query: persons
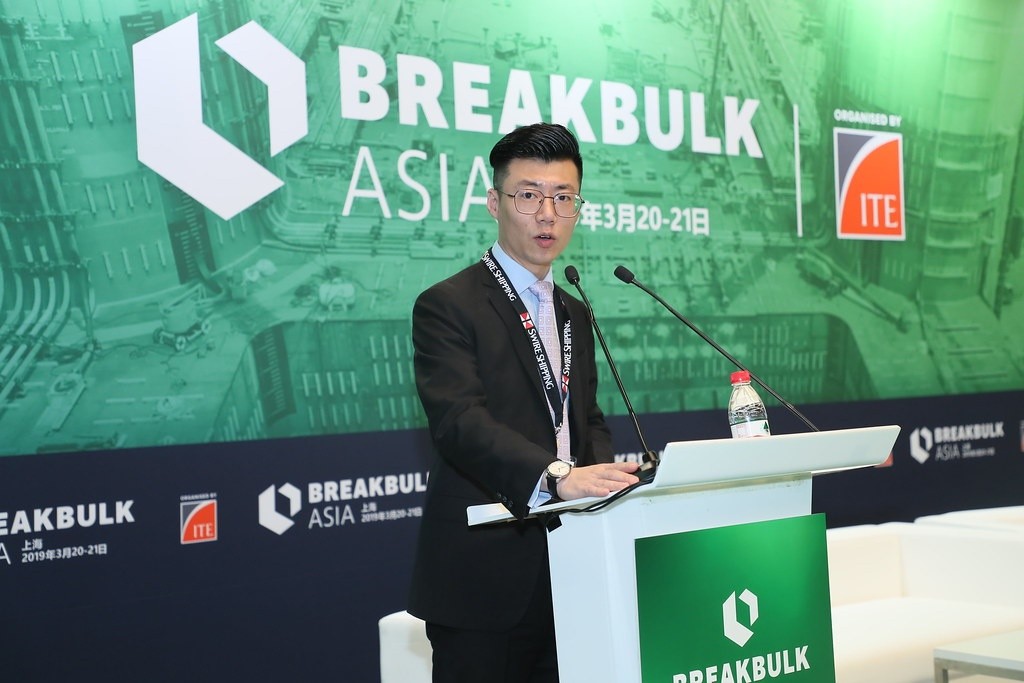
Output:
[405,125,639,683]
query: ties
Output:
[528,281,562,400]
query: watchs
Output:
[546,460,572,500]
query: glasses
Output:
[495,188,586,218]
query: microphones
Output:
[614,265,819,432]
[565,265,661,483]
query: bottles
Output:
[727,371,771,438]
